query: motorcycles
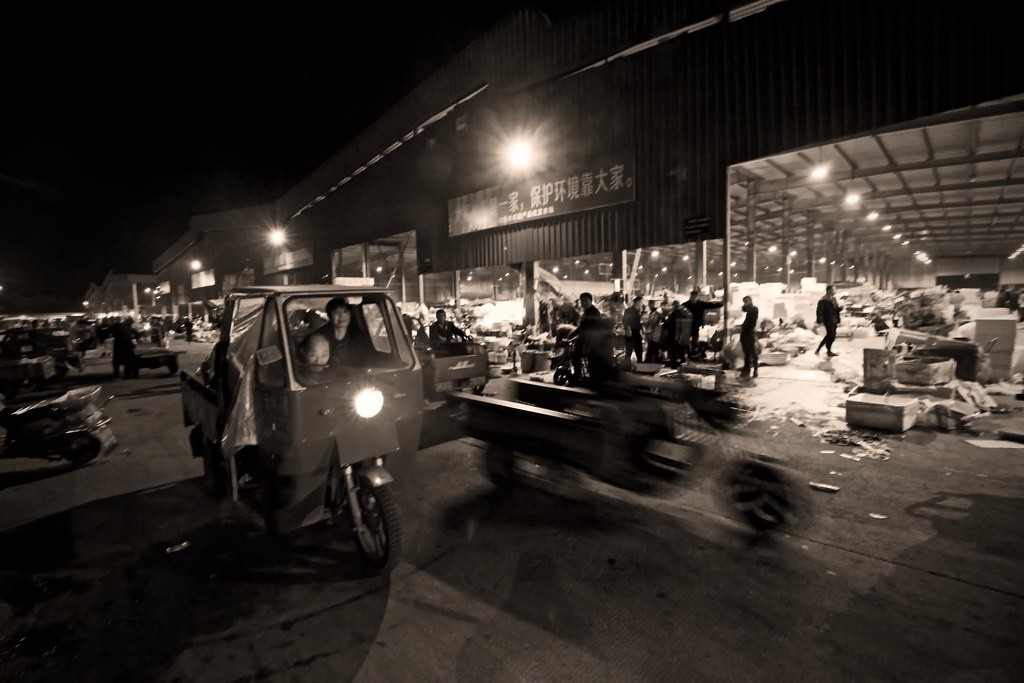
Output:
[177,283,428,574]
[127,326,190,379]
[370,314,492,401]
[0,383,120,466]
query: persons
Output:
[622,290,726,369]
[1,313,101,352]
[552,293,603,387]
[872,294,890,336]
[296,297,366,383]
[110,316,174,379]
[814,285,844,356]
[184,316,193,342]
[996,288,1024,323]
[430,310,466,356]
[735,296,758,379]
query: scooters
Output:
[548,342,637,400]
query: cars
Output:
[0,327,78,404]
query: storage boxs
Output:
[844,287,1019,430]
